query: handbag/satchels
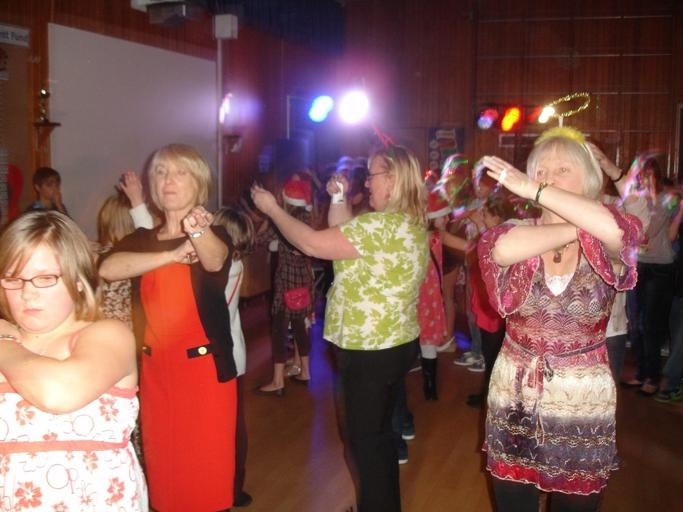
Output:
[283,286,312,310]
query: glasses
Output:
[365,169,388,182]
[0,272,62,290]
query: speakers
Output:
[215,14,237,40]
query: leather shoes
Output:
[620,379,643,389]
[638,386,660,398]
[253,364,310,397]
[234,491,252,506]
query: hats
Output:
[282,171,316,213]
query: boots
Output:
[418,355,438,402]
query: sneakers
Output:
[453,351,483,367]
[654,386,682,404]
[397,446,408,465]
[466,358,486,372]
[400,421,415,441]
